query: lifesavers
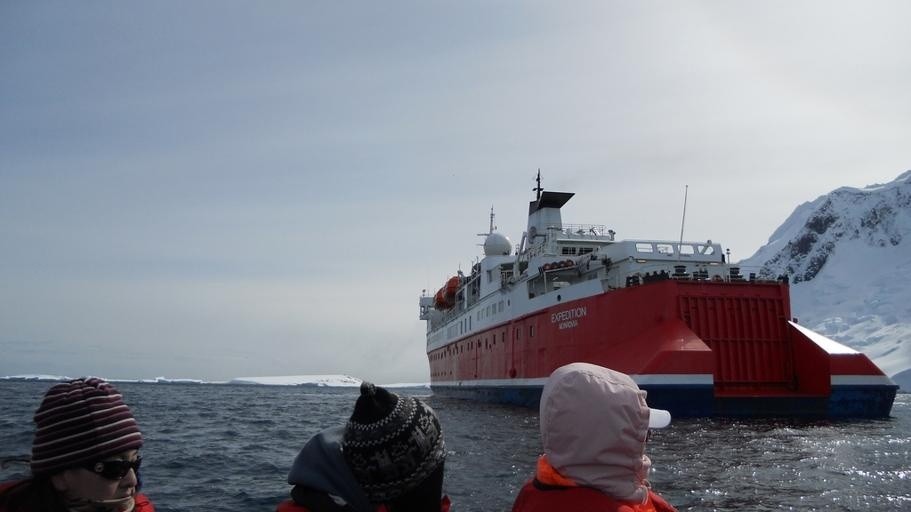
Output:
[711,275,722,281]
[545,259,574,270]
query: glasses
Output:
[80,457,143,481]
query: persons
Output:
[0,374,155,510]
[513,361,679,511]
[285,380,453,512]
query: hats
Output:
[29,375,144,478]
[341,386,448,503]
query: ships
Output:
[419,168,900,418]
